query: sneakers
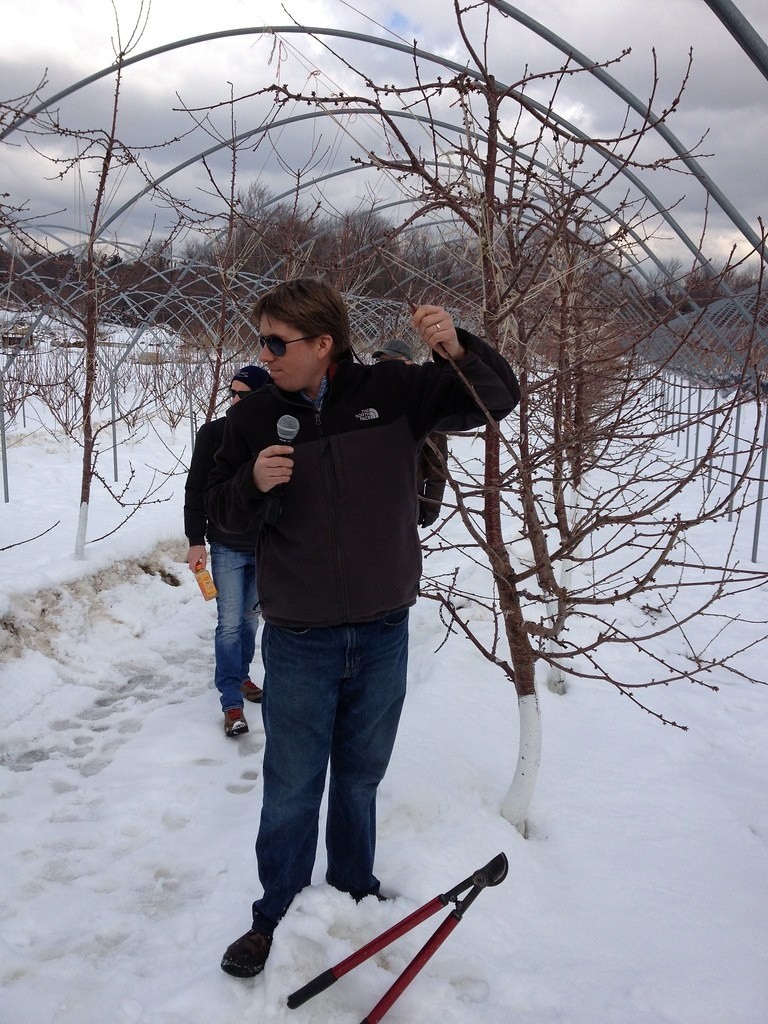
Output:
[224,707,249,738]
[221,913,274,978]
[326,872,386,903]
[240,680,263,703]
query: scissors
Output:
[285,852,512,1024]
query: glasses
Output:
[228,388,251,400]
[259,332,319,356]
[375,358,410,364]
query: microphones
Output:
[265,415,300,525]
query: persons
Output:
[372,339,448,529]
[201,279,519,977]
[184,366,274,736]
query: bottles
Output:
[195,563,218,602]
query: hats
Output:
[230,365,275,393]
[371,340,414,363]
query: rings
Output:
[435,323,441,333]
